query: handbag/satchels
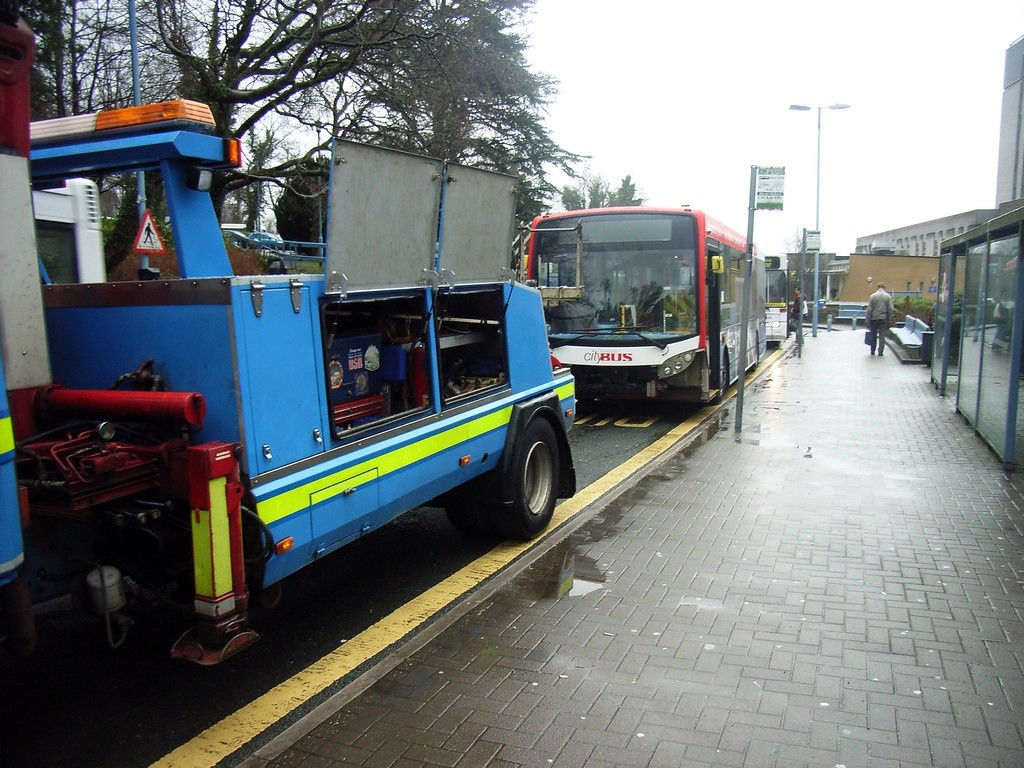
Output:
[865,327,873,345]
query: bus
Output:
[523,205,767,406]
[764,254,788,347]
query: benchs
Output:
[833,305,867,325]
[889,314,932,360]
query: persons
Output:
[830,285,839,298]
[865,282,893,355]
[791,286,809,346]
[991,285,1009,352]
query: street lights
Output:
[788,104,852,339]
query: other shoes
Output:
[879,353,884,357]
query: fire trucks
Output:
[0,0,578,668]
